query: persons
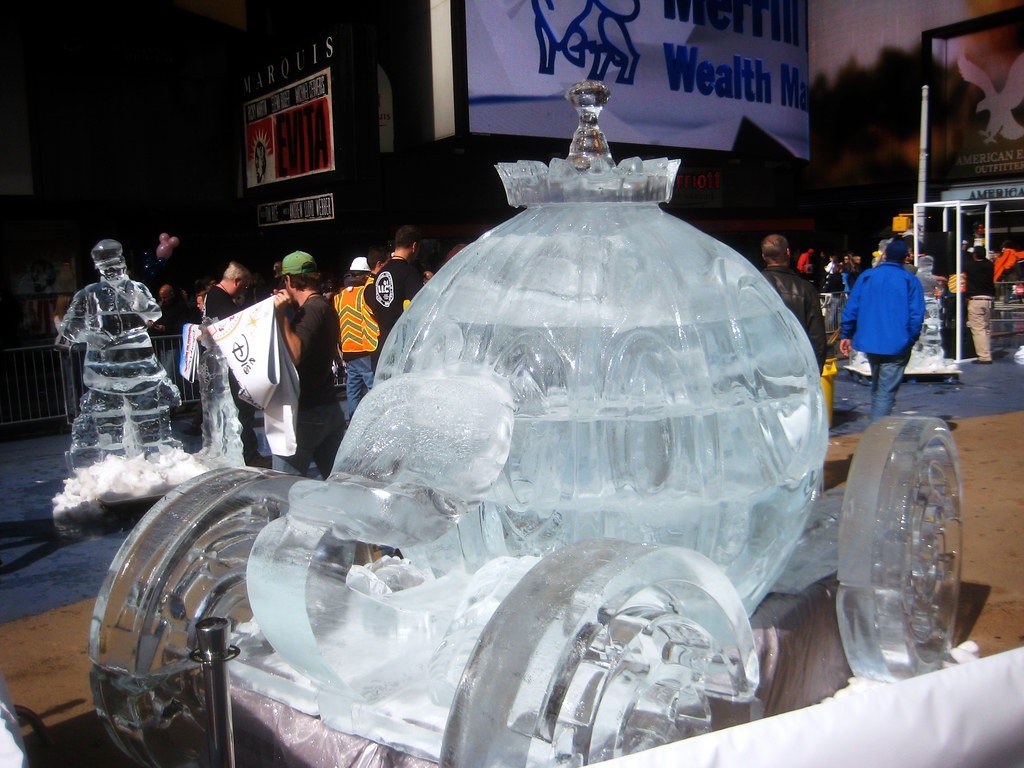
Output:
[206,262,271,466]
[798,241,995,363]
[375,225,421,362]
[758,235,826,377]
[840,241,925,425]
[271,251,347,481]
[334,257,375,420]
[147,246,434,437]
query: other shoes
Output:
[180,427,202,435]
[972,360,992,364]
[246,453,272,468]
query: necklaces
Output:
[220,283,228,294]
[307,293,322,298]
[392,256,406,261]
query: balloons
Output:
[131,233,180,279]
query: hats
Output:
[886,240,909,259]
[275,250,317,278]
[350,257,371,271]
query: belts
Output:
[970,298,991,300]
